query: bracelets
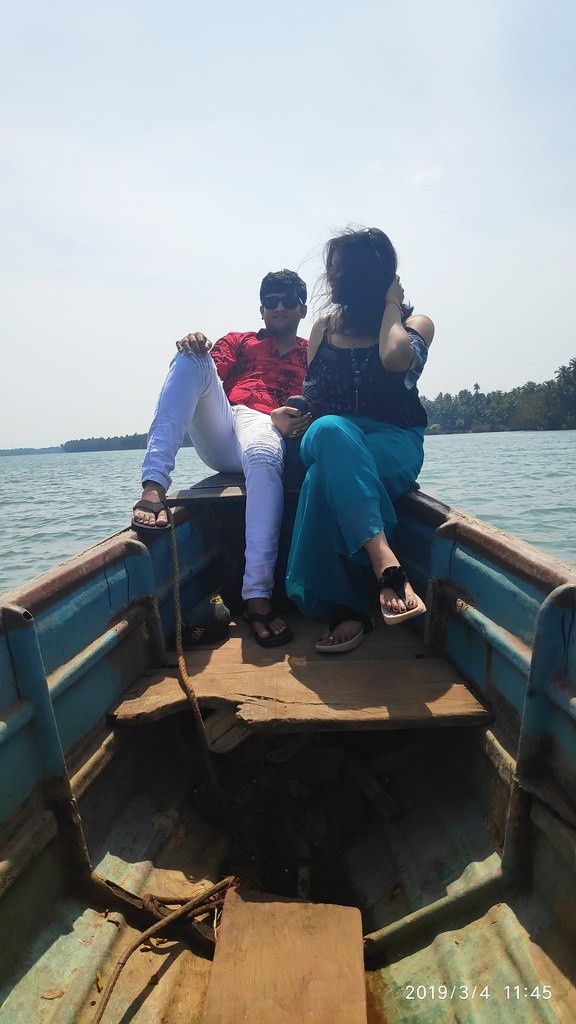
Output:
[385,301,404,319]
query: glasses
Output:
[263,295,304,309]
[355,226,386,270]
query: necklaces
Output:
[345,327,375,416]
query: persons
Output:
[272,227,436,655]
[131,269,310,649]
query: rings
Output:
[293,429,298,435]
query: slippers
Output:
[377,565,427,625]
[315,612,375,652]
[242,608,292,648]
[131,499,172,533]
